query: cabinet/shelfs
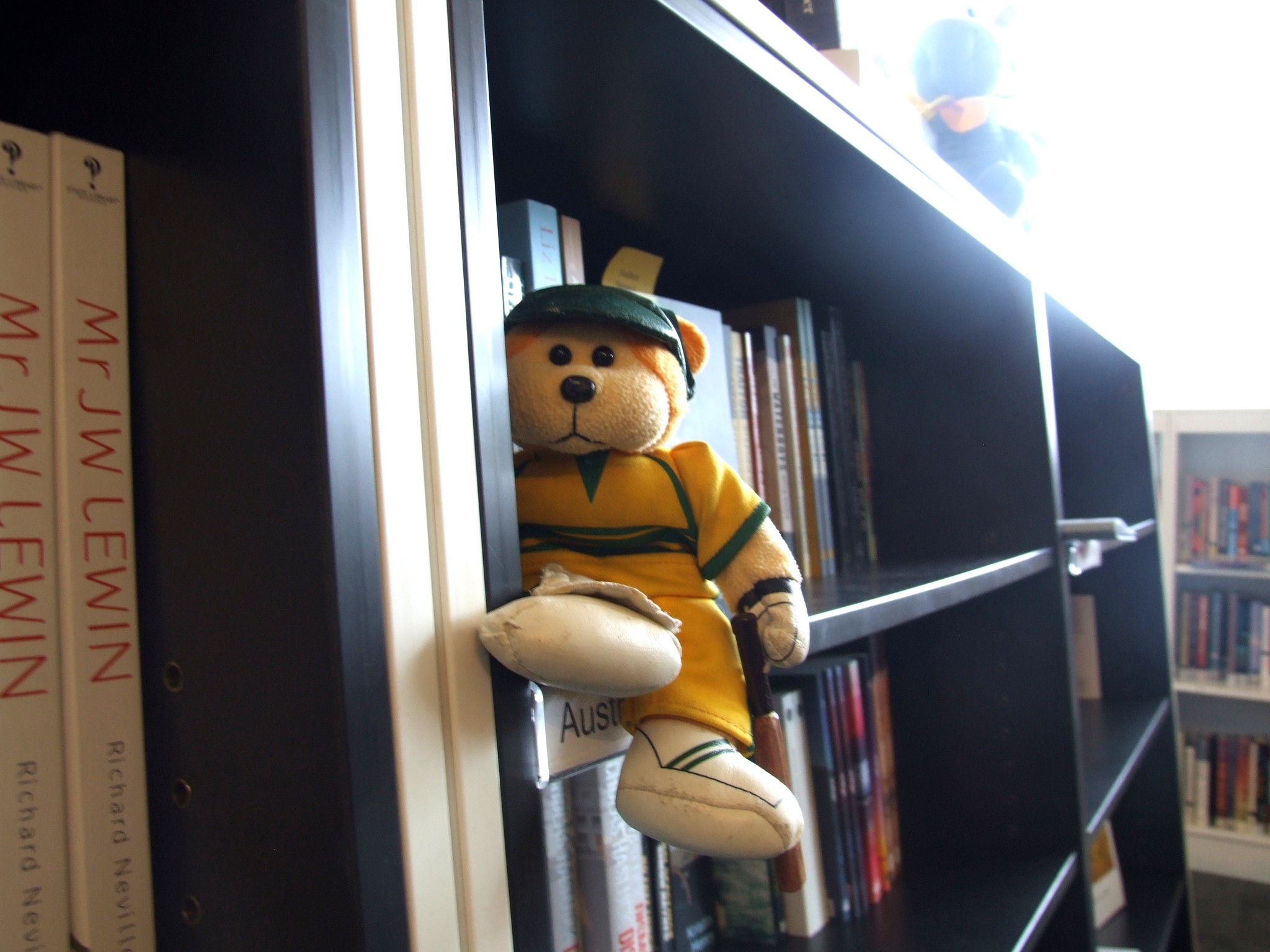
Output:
[0,0,1270,952]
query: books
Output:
[1179,589,1270,680]
[1089,820,1126,928]
[1183,731,1270,836]
[0,120,156,952]
[1070,596,1103,701]
[1180,476,1270,564]
[539,756,789,952]
[753,653,900,938]
[497,199,874,581]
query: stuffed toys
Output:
[478,283,810,859]
[910,8,1038,217]
